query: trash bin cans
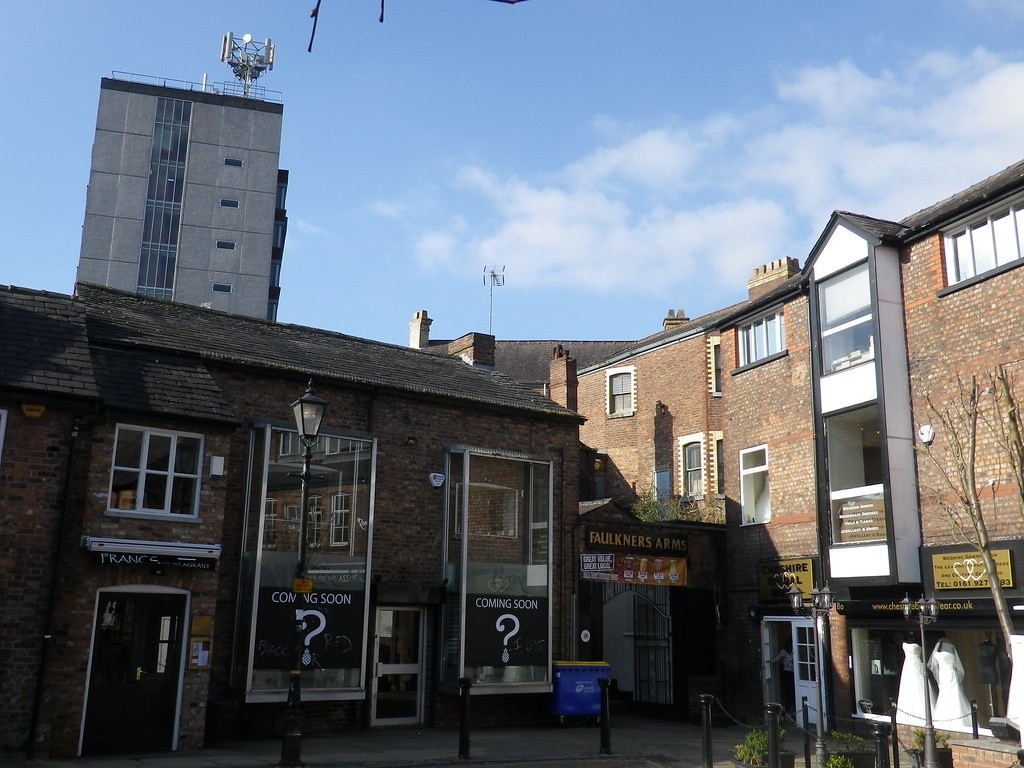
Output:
[552,661,612,727]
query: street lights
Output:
[279,377,329,768]
[788,578,836,768]
[902,590,940,768]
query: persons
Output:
[977,640,1000,683]
[895,632,936,725]
[927,638,980,728]
[768,636,795,712]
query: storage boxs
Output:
[837,500,887,540]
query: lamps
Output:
[988,716,1020,742]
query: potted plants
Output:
[723,728,876,768]
[905,728,955,768]
